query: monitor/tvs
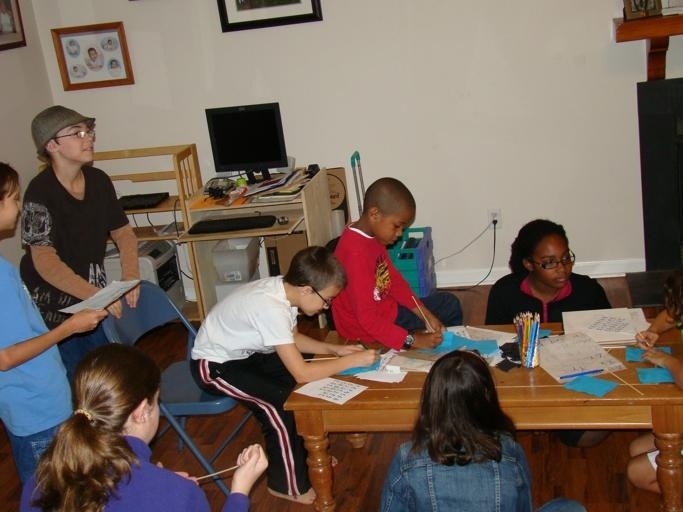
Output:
[204,101,288,185]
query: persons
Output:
[485,220,611,448]
[0,161,109,487]
[381,348,587,512]
[189,245,379,501]
[623,266,683,494]
[20,344,268,512]
[18,106,140,333]
[331,177,461,348]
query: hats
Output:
[32,105,95,156]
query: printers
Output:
[102,239,181,293]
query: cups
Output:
[517,339,542,368]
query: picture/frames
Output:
[50,22,135,91]
[623,0,663,22]
[0,0,28,51]
[217,0,324,33]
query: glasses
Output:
[531,249,576,268]
[51,130,94,138]
[302,286,331,309]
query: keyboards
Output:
[188,215,276,235]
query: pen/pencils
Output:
[636,327,651,348]
[560,369,603,379]
[513,311,540,368]
[357,339,370,350]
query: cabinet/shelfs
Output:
[38,143,203,324]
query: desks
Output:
[184,167,334,330]
[282,328,683,512]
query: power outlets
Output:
[487,208,503,228]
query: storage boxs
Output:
[214,272,260,302]
[209,236,259,282]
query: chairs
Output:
[100,278,255,498]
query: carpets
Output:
[624,271,669,308]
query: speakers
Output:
[277,156,296,172]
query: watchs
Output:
[403,331,414,349]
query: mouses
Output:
[278,216,289,225]
